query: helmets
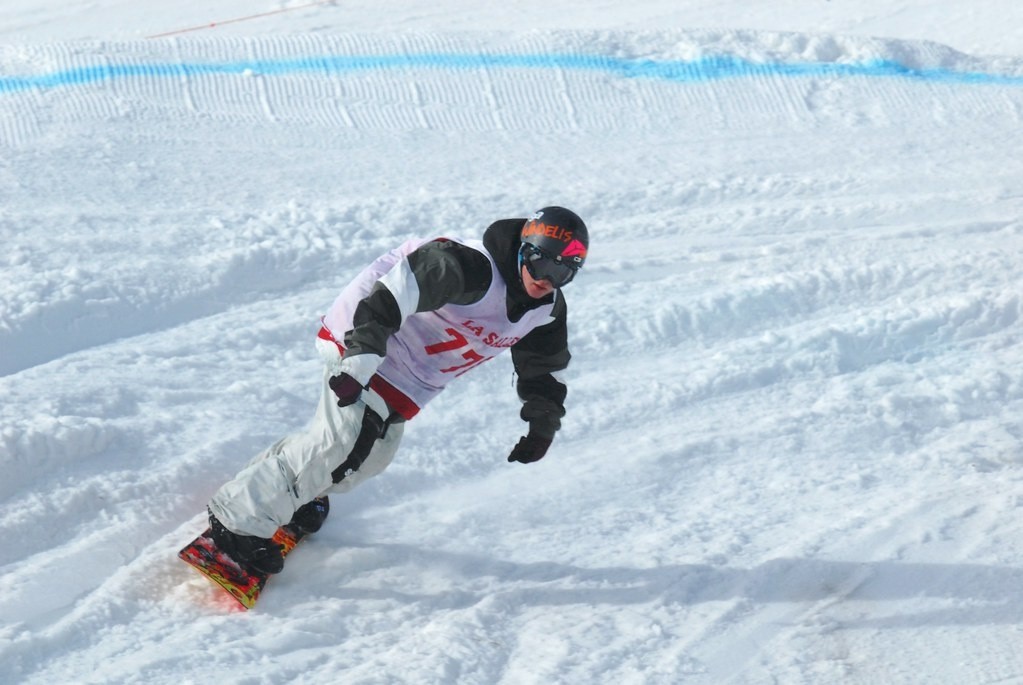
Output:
[519,206,589,268]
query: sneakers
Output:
[290,493,330,534]
[209,512,284,575]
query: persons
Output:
[205,206,588,576]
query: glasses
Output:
[516,242,577,289]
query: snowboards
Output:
[175,494,332,613]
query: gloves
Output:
[328,373,364,407]
[508,432,552,465]
